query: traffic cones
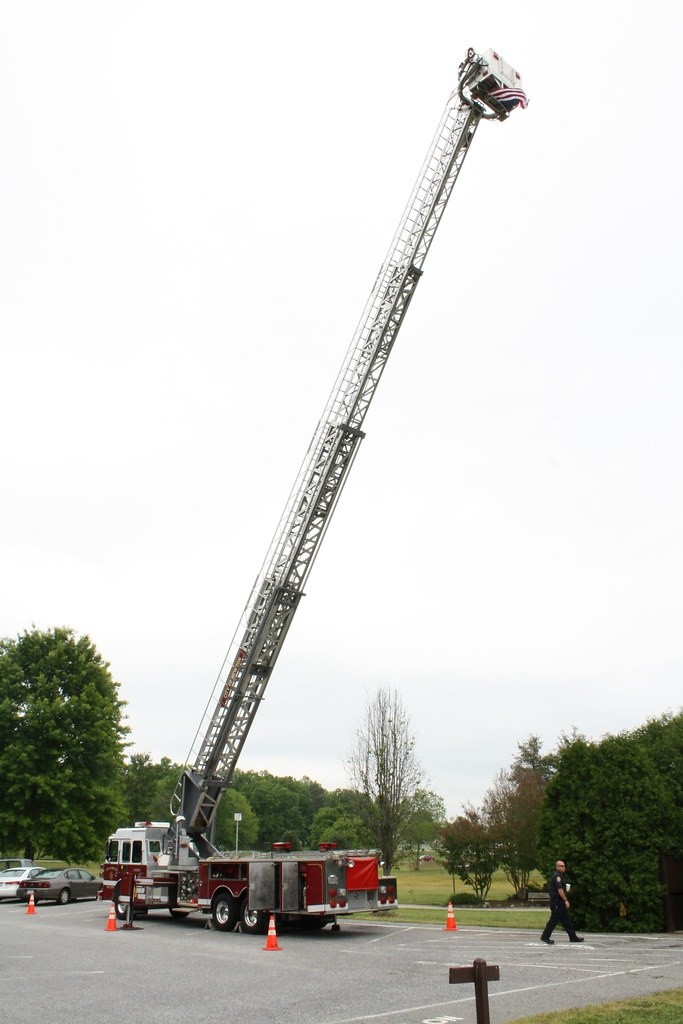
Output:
[104,903,118,931]
[262,915,283,951]
[24,892,37,915]
[442,902,459,931]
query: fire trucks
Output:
[92,42,530,936]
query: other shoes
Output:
[540,937,554,945]
[570,935,584,942]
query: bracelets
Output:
[564,899,568,901]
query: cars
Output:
[17,868,104,904]
[419,855,436,862]
[0,867,47,902]
[347,849,377,856]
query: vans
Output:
[0,859,36,872]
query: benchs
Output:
[528,892,550,907]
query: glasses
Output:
[558,865,565,867]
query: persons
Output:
[540,861,584,945]
